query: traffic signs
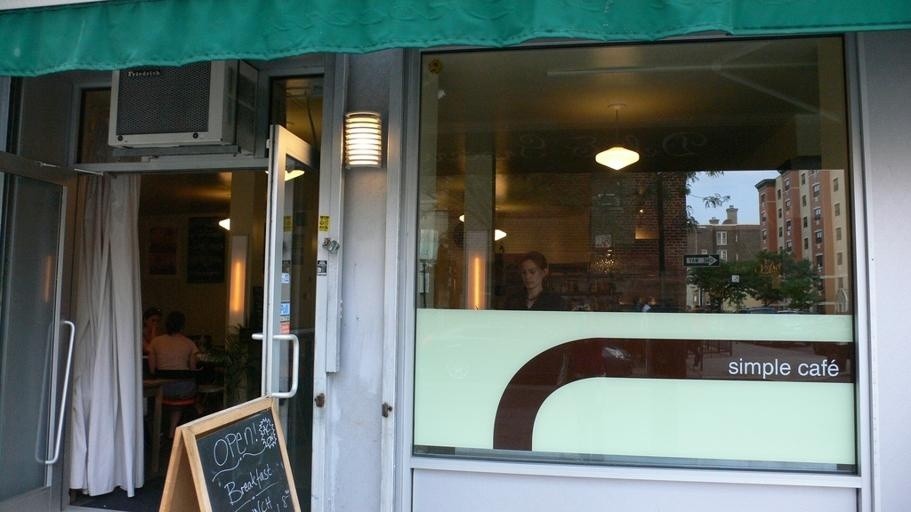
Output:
[684,253,720,268]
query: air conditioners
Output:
[108,56,259,157]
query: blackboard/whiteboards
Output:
[182,395,304,512]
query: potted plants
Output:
[685,251,827,312]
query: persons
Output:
[498,250,567,310]
[142,304,206,442]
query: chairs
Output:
[143,356,223,446]
